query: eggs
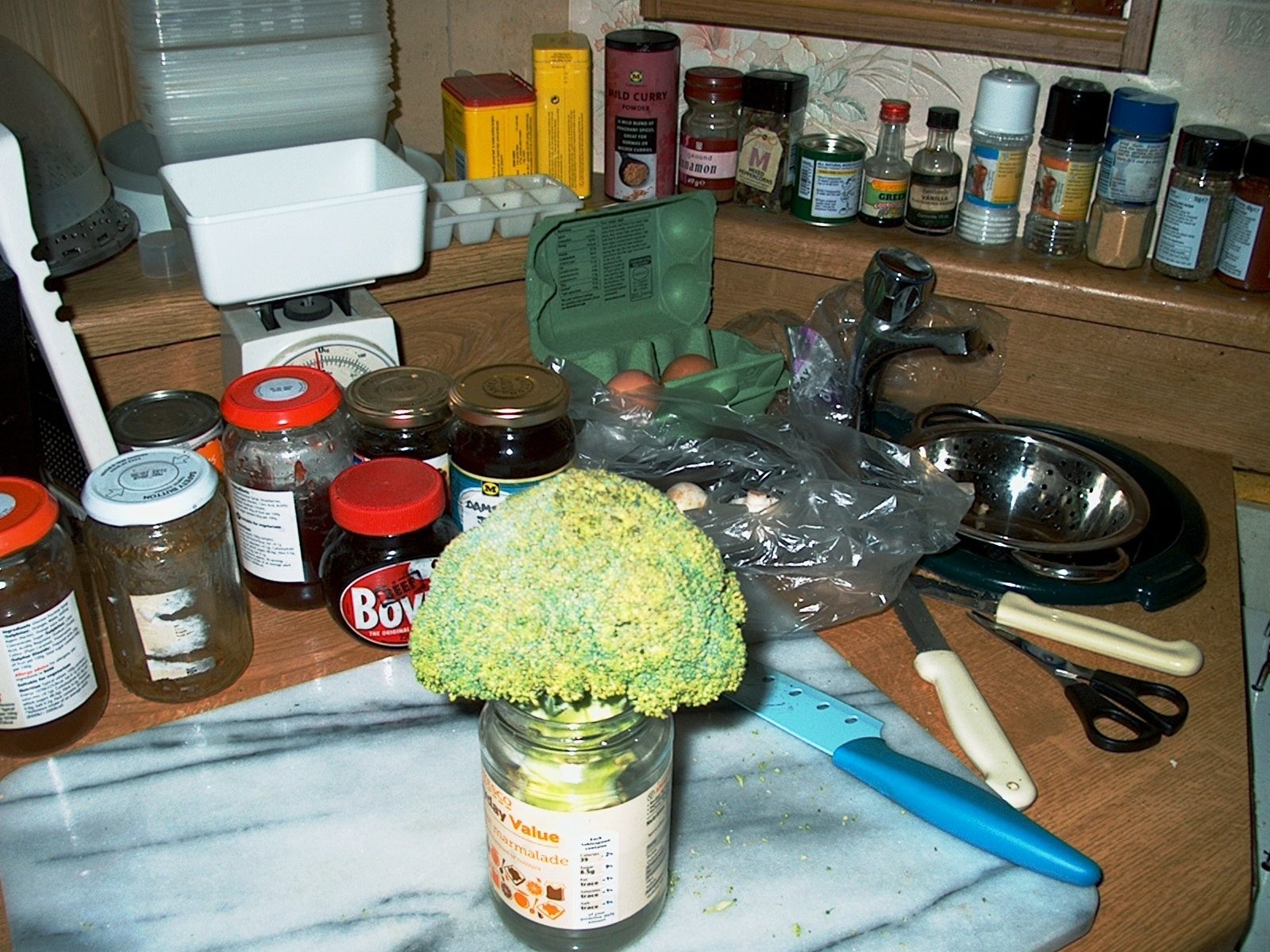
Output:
[603,350,718,416]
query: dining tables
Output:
[0,419,1263,948]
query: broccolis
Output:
[410,461,748,823]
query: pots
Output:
[903,403,1152,583]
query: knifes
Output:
[909,572,1206,676]
[892,578,1037,811]
[721,659,1105,887]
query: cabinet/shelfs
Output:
[63,170,1269,478]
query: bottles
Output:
[857,67,1270,291]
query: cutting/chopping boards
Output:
[3,566,1101,952]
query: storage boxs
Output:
[120,0,421,306]
